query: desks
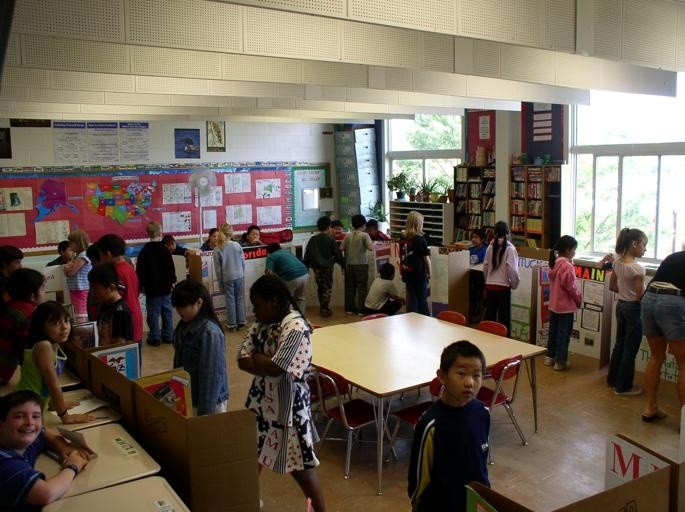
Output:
[307,312,548,495]
[0,356,194,512]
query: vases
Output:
[448,189,454,202]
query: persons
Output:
[1,211,431,512]
[481,219,522,339]
[406,338,491,512]
[605,227,648,396]
[638,248,685,423]
[465,231,489,264]
[543,235,583,372]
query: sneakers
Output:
[553,360,571,370]
[614,388,641,395]
[224,324,237,329]
[543,356,554,366]
[320,309,331,318]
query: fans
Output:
[187,166,217,248]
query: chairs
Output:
[307,378,336,428]
[475,355,528,466]
[478,321,508,337]
[383,377,444,463]
[437,310,466,326]
[315,366,398,479]
[361,314,388,321]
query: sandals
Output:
[642,410,667,422]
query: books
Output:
[452,161,550,248]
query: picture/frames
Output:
[290,162,331,233]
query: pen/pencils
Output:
[96,416,109,419]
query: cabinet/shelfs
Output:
[389,201,454,246]
[508,164,573,249]
[453,165,495,247]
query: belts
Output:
[647,285,681,295]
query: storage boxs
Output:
[334,128,380,221]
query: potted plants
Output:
[388,173,410,199]
[406,188,417,201]
[439,186,447,202]
[431,191,440,202]
[417,177,436,202]
[366,198,389,236]
